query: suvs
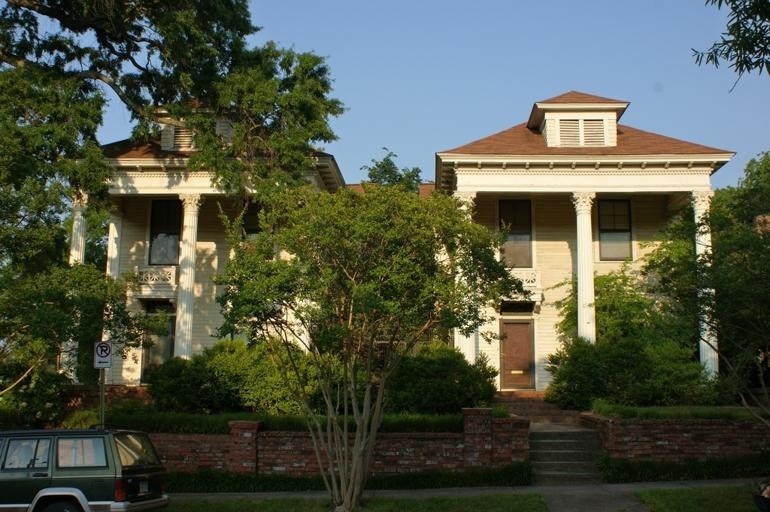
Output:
[0,428,171,512]
